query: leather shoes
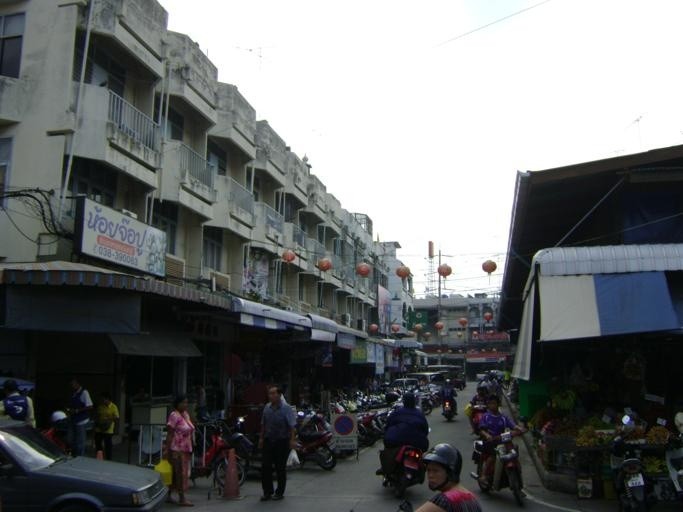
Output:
[262,493,283,501]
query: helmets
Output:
[445,379,451,385]
[423,443,462,482]
[51,411,67,422]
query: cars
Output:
[0,416,170,511]
[476,370,505,382]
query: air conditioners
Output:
[202,266,231,296]
[144,253,186,288]
[318,307,328,317]
[276,293,291,307]
[298,301,312,314]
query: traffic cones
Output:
[95,449,104,461]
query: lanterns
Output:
[282,251,498,353]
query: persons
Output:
[376,391,429,474]
[215,382,225,409]
[1,373,149,460]
[259,384,298,501]
[165,395,195,505]
[438,370,521,482]
[197,382,206,418]
[413,442,480,511]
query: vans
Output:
[419,363,465,391]
[402,372,443,382]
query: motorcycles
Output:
[655,415,681,511]
[438,388,458,422]
[471,416,528,509]
[288,378,442,458]
[252,422,336,470]
[466,383,504,438]
[348,501,414,512]
[596,413,649,511]
[195,414,278,481]
[139,412,247,489]
[377,402,430,498]
